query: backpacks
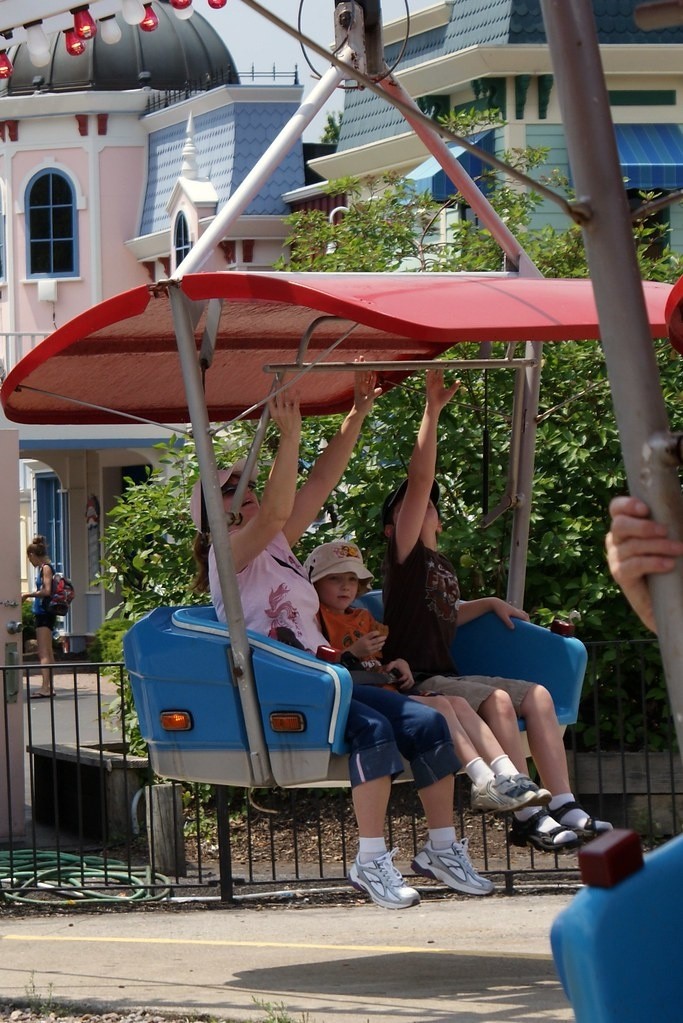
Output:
[40,563,74,616]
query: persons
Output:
[603,273,683,634]
[21,535,58,699]
[188,354,497,910]
[378,368,613,856]
[302,539,553,812]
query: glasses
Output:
[222,481,256,496]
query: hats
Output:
[303,541,375,600]
[190,458,258,534]
[382,477,440,524]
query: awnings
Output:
[567,123,683,194]
[396,129,495,206]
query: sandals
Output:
[545,801,609,840]
[508,811,582,852]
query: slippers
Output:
[30,691,56,698]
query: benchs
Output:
[26,744,151,844]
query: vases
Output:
[66,635,86,653]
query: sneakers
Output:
[411,838,494,895]
[470,775,536,814]
[512,774,552,812]
[348,847,420,909]
